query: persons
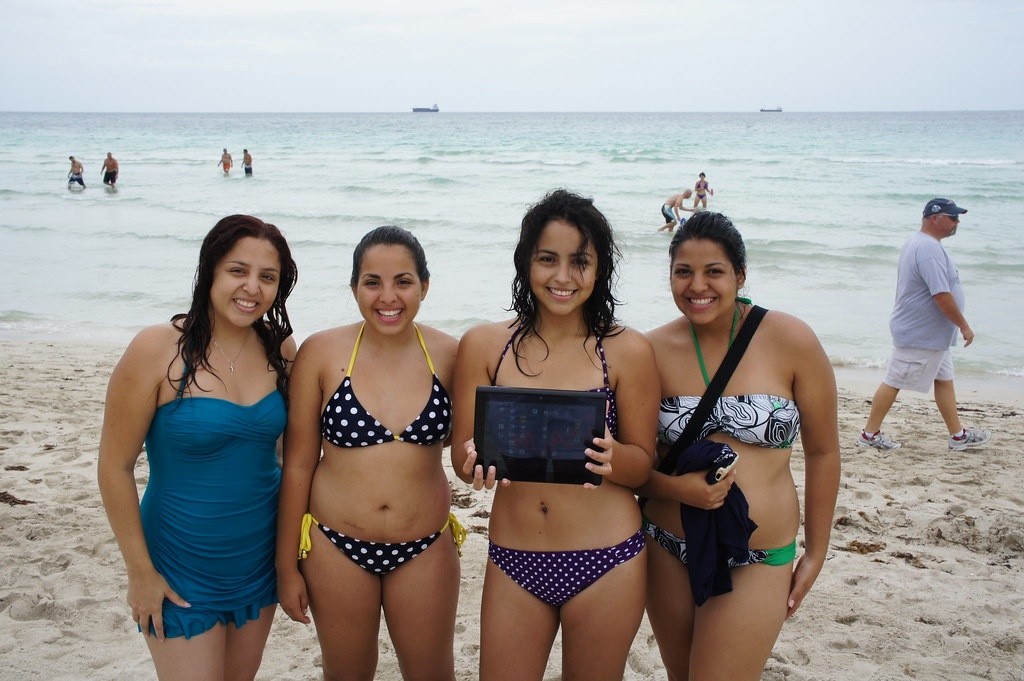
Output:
[218,148,232,174]
[451,187,661,681]
[693,172,714,208]
[100,152,118,188]
[856,198,994,452]
[98,215,298,681]
[643,213,838,681]
[241,149,252,175]
[68,156,87,189]
[277,227,462,681]
[658,189,695,232]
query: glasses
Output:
[942,213,959,220]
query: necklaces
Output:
[212,327,252,372]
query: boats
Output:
[412,104,440,112]
[760,107,782,112]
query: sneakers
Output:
[857,429,901,450]
[948,427,991,449]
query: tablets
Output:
[472,386,607,487]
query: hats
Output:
[922,198,968,217]
